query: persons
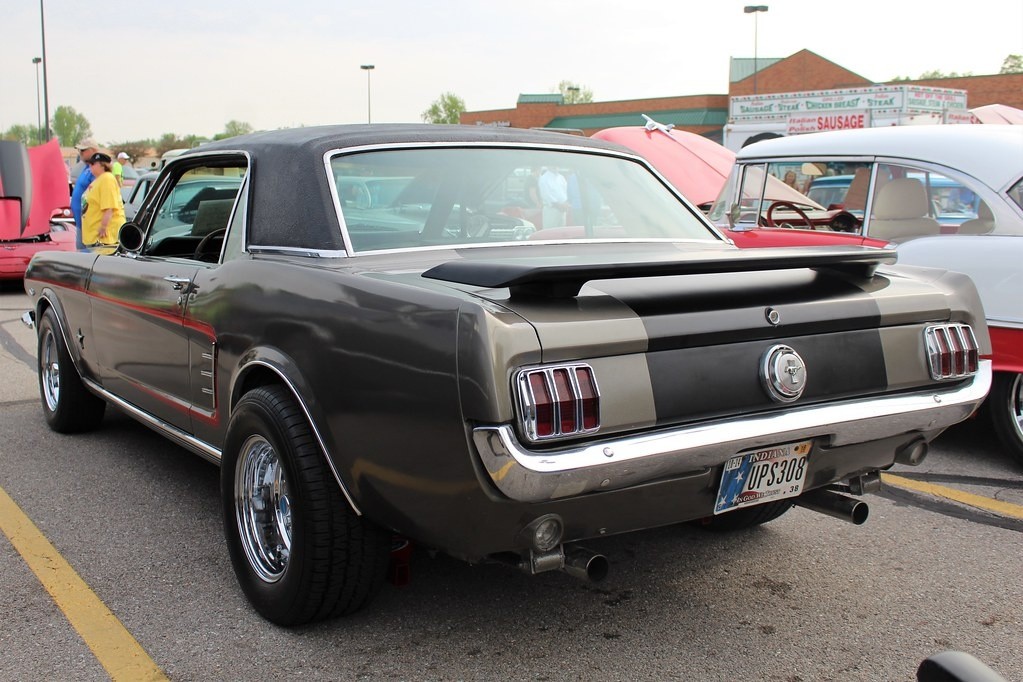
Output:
[523,162,573,228]
[69,139,96,250]
[782,170,811,195]
[110,151,131,204]
[81,154,127,248]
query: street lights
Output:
[31,56,42,144]
[744,4,770,97]
[360,65,375,124]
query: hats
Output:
[85,153,110,163]
[75,143,98,150]
[118,152,129,159]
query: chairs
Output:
[956,197,995,237]
[867,178,941,245]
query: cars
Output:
[22,125,992,638]
[0,134,80,278]
[533,106,1023,456]
[61,146,534,250]
